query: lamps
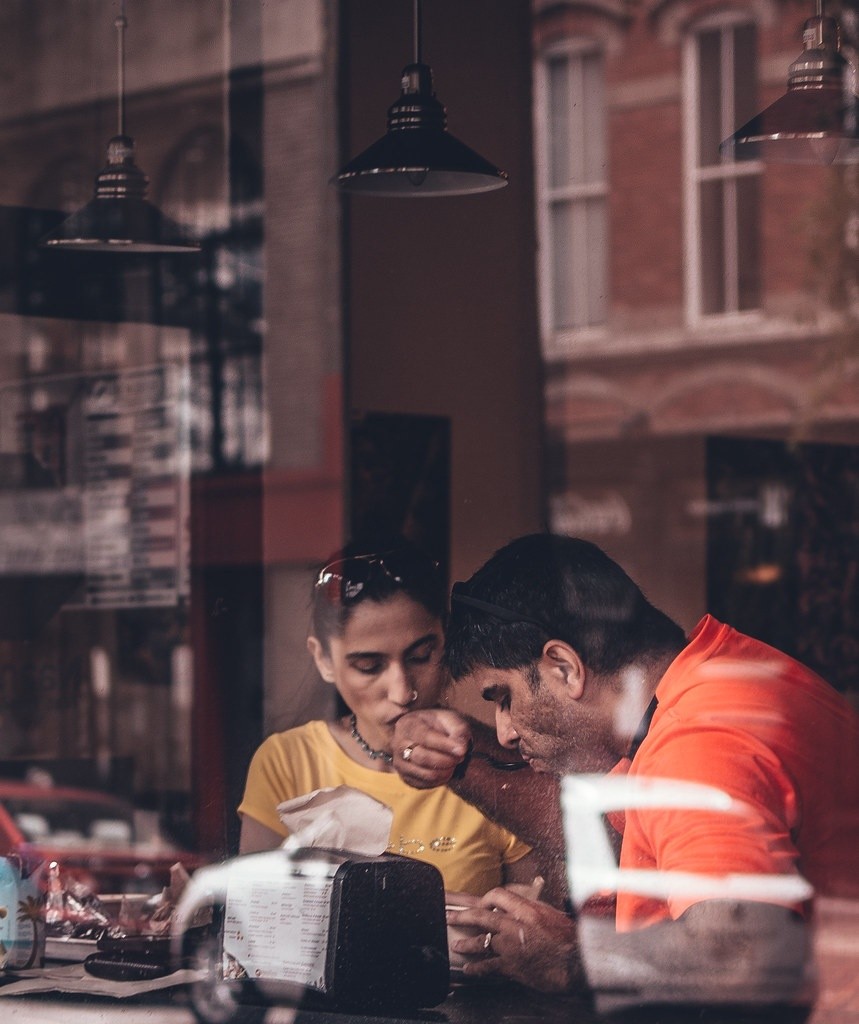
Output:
[716,1,859,164]
[326,1,509,198]
[43,1,203,252]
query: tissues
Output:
[211,782,450,1012]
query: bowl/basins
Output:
[445,905,498,991]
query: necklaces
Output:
[349,713,392,764]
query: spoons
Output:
[470,753,530,771]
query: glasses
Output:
[450,581,586,663]
[312,550,449,603]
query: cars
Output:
[0,780,209,941]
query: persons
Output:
[391,533,859,1024]
[233,529,538,905]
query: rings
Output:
[401,743,418,759]
[483,931,493,949]
